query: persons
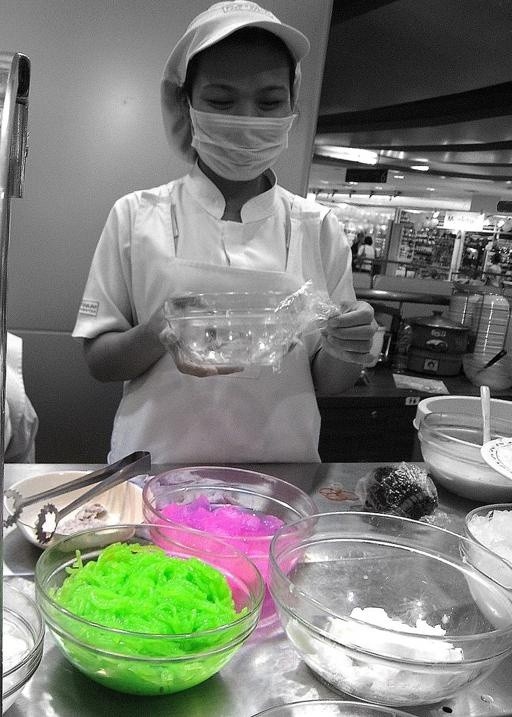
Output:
[349,229,366,272]
[356,235,377,274]
[482,251,502,288]
[71,0,382,466]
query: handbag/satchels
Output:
[358,245,367,265]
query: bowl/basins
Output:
[462,353,512,392]
[0,580,44,713]
[266,511,512,709]
[457,502,512,633]
[418,410,512,502]
[451,293,511,356]
[2,470,156,551]
[33,520,264,695]
[143,465,317,632]
[163,294,298,375]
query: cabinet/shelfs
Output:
[317,397,416,463]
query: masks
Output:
[185,92,294,182]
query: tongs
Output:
[2,449,150,541]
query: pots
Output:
[402,309,472,354]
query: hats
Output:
[160,0,310,166]
